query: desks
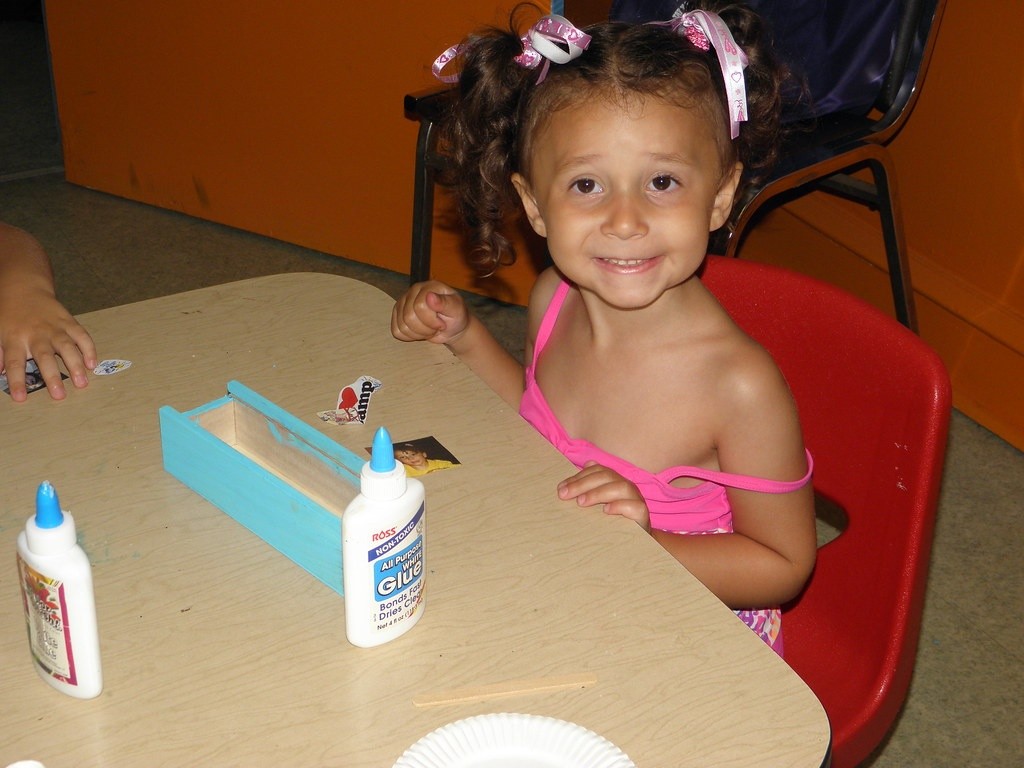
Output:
[0,272,834,768]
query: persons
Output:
[0,222,98,403]
[393,441,453,477]
[391,1,817,657]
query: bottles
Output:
[340,426,425,648]
[16,479,102,700]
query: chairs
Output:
[402,0,946,338]
[688,254,949,768]
